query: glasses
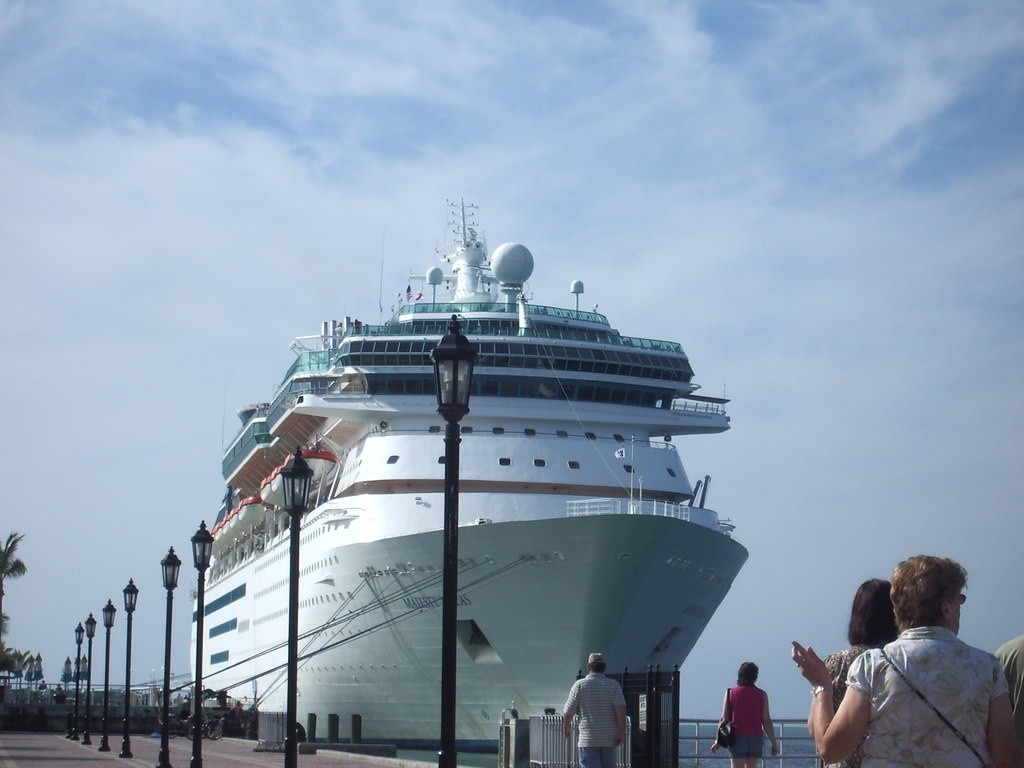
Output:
[948,594,967,608]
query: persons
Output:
[563,653,627,768]
[710,662,779,768]
[232,701,244,717]
[790,554,1024,768]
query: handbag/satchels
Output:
[717,688,732,748]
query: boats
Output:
[206,448,337,558]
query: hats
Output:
[588,653,605,663]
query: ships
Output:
[183,198,749,768]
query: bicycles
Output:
[167,711,225,741]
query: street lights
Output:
[97,598,118,752]
[70,621,85,741]
[80,612,97,745]
[278,444,315,768]
[119,577,141,758]
[190,519,215,768]
[426,316,485,768]
[153,546,184,768]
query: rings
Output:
[797,664,800,667]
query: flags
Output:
[416,293,424,300]
[406,286,412,301]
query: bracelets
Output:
[815,686,833,696]
